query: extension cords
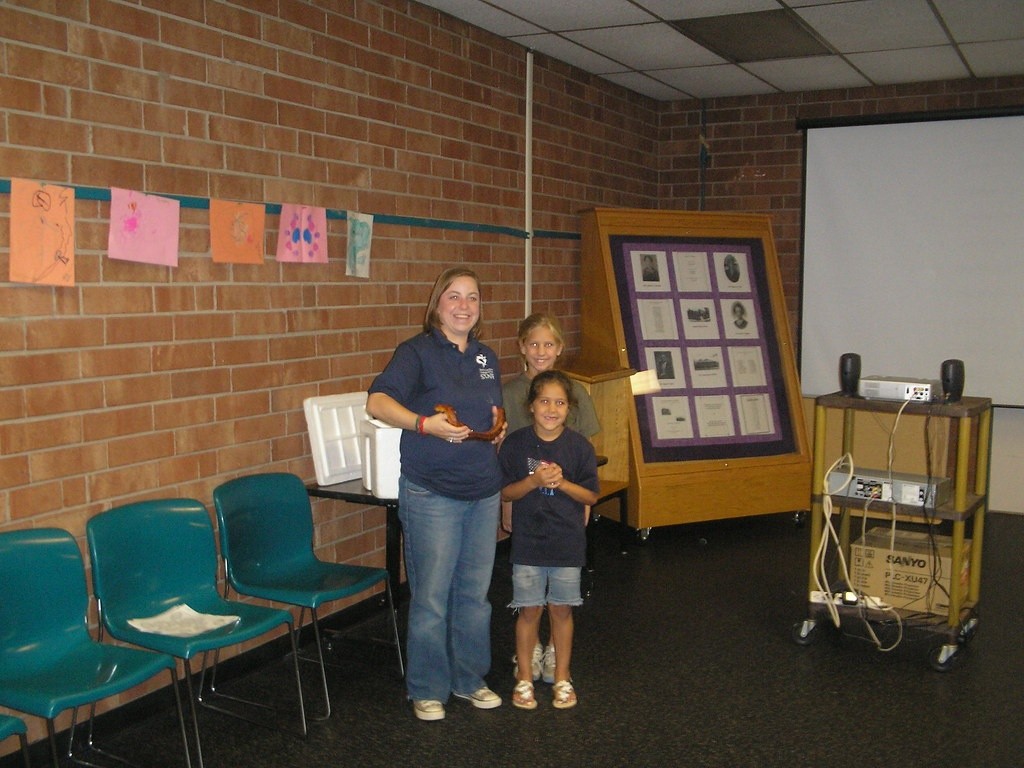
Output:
[809,591,881,609]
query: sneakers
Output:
[453,686,502,709]
[540,646,556,683]
[413,699,445,720]
[512,644,543,681]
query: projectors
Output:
[856,373,944,404]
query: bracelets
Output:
[419,416,427,434]
[416,416,420,434]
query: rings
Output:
[450,438,453,443]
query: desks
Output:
[302,454,607,651]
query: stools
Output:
[591,479,628,554]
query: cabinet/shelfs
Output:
[792,388,992,671]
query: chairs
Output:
[209,472,406,722]
[0,528,195,768]
[85,497,310,768]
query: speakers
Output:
[840,353,861,399]
[941,359,965,403]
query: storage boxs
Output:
[359,418,402,499]
[849,528,974,615]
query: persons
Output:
[733,303,748,329]
[366,267,603,720]
[725,258,739,281]
[658,354,672,377]
[643,256,659,280]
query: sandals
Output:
[551,679,577,709]
[512,680,538,709]
